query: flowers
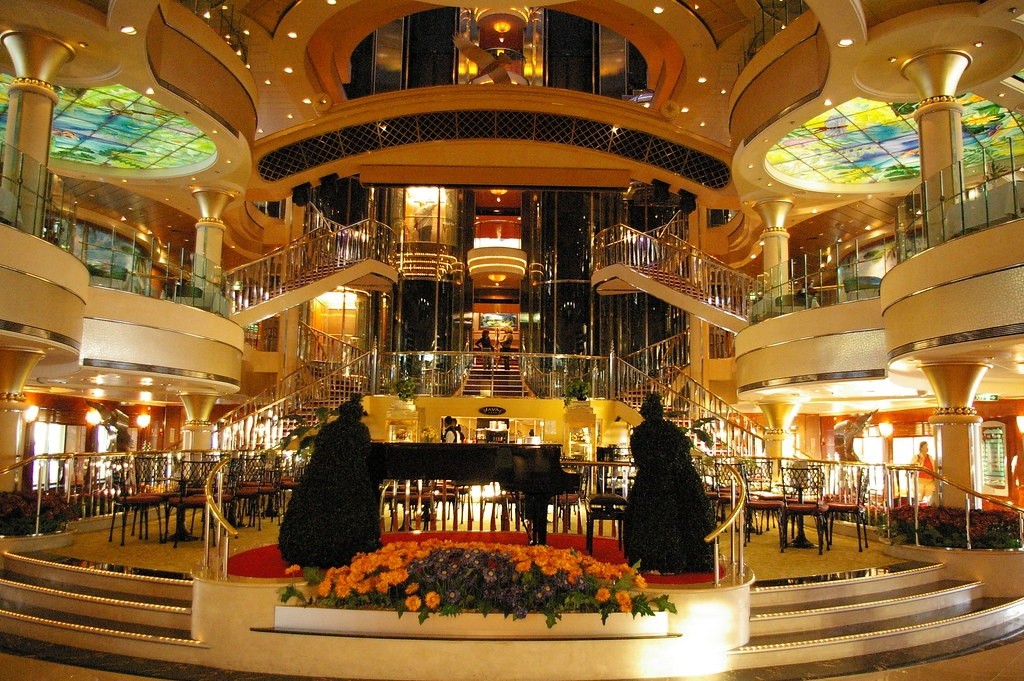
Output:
[0,489,80,536]
[275,538,677,629]
[875,502,1023,549]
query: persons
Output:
[443,416,464,443]
[476,330,494,370]
[912,442,938,505]
[498,326,513,370]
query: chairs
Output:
[109,450,869,556]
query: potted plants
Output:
[621,393,714,577]
[394,377,417,402]
[563,381,591,407]
